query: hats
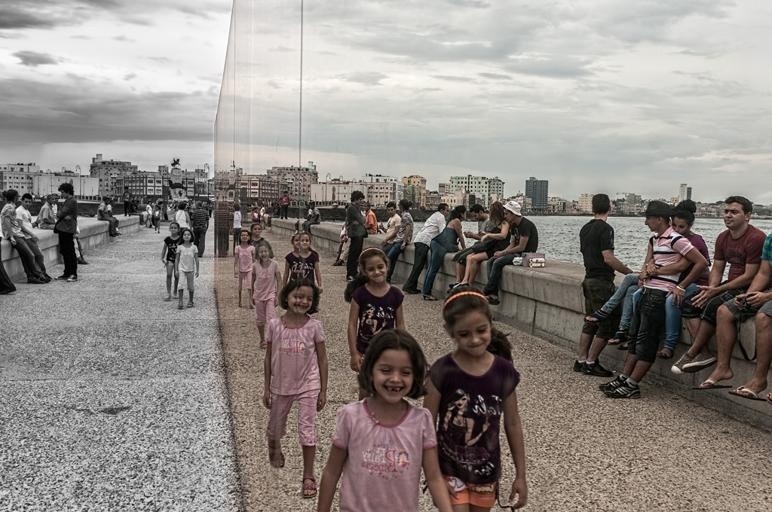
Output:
[645,201,677,217]
[503,201,522,216]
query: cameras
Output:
[738,296,758,313]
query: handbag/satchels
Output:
[472,238,496,252]
[522,252,545,266]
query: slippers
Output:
[728,386,772,405]
[691,378,732,389]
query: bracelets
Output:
[675,283,687,293]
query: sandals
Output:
[584,309,608,322]
[268,443,285,467]
[163,294,193,309]
[260,339,266,349]
[656,346,673,359]
[607,330,631,350]
[302,477,317,498]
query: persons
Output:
[345,247,407,373]
[0,182,540,310]
[423,282,531,511]
[670,195,768,376]
[599,199,709,400]
[617,199,711,359]
[764,391,771,404]
[249,238,284,350]
[316,326,455,512]
[571,191,635,378]
[584,273,641,345]
[262,275,330,499]
[699,231,771,405]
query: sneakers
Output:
[332,257,359,282]
[682,350,716,372]
[77,255,89,264]
[446,282,501,305]
[402,285,438,300]
[599,374,627,391]
[573,359,613,377]
[27,272,77,284]
[671,353,692,375]
[604,380,640,399]
